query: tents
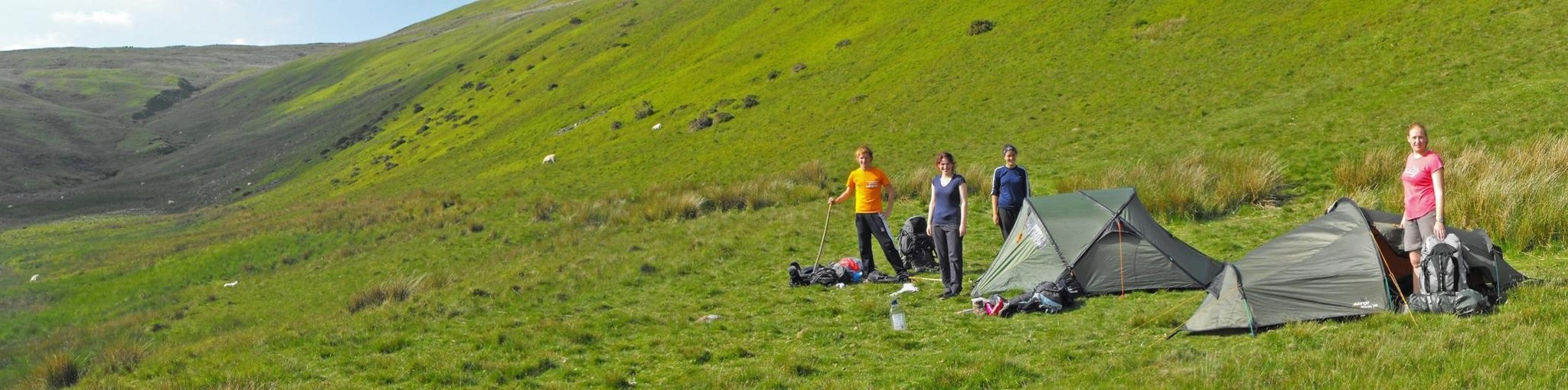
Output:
[973,189,1226,294]
[1169,197,1547,342]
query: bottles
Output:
[989,299,1004,316]
[889,300,907,333]
[985,302,993,315]
[852,271,863,282]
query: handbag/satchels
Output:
[1408,288,1491,315]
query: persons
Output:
[991,144,1031,243]
[926,152,968,300]
[828,146,910,284]
[1401,122,1448,293]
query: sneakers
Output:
[939,291,959,299]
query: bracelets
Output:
[1435,219,1444,223]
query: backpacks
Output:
[898,216,935,270]
[1419,233,1470,295]
